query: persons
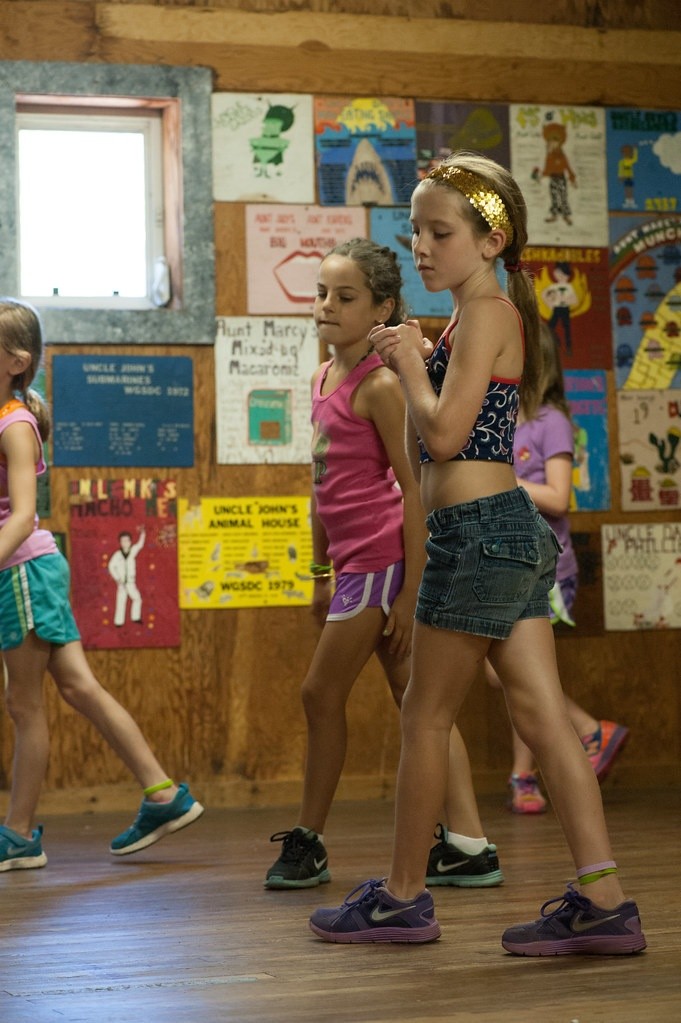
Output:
[262,239,504,888]
[508,314,630,816]
[0,297,204,869]
[312,153,647,960]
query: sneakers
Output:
[509,772,550,813]
[580,720,630,781]
[105,782,205,858]
[0,824,50,872]
[423,829,505,888]
[310,877,441,944]
[266,829,333,889]
[502,890,647,957]
